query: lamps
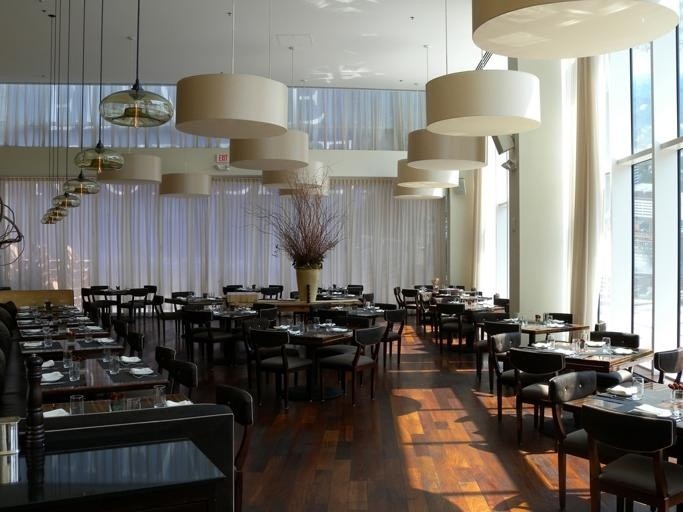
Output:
[393,83,444,199]
[51,3,81,209]
[229,4,309,171]
[74,4,124,171]
[63,2,100,194]
[397,50,458,188]
[99,42,161,183]
[278,83,330,198]
[40,14,68,224]
[408,3,485,170]
[426,3,542,137]
[262,51,326,187]
[158,84,209,198]
[175,4,288,139]
[471,2,679,59]
[99,2,173,127]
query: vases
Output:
[296,268,320,300]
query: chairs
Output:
[0,283,404,415]
[475,311,682,510]
[25,404,233,511]
[402,284,510,361]
[212,381,255,512]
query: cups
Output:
[0,454,19,484]
[69,394,85,414]
[631,377,645,400]
[183,283,380,341]
[153,384,166,408]
[507,312,627,360]
[122,397,140,409]
[15,300,152,382]
[421,273,495,314]
[0,422,19,455]
[670,389,682,421]
